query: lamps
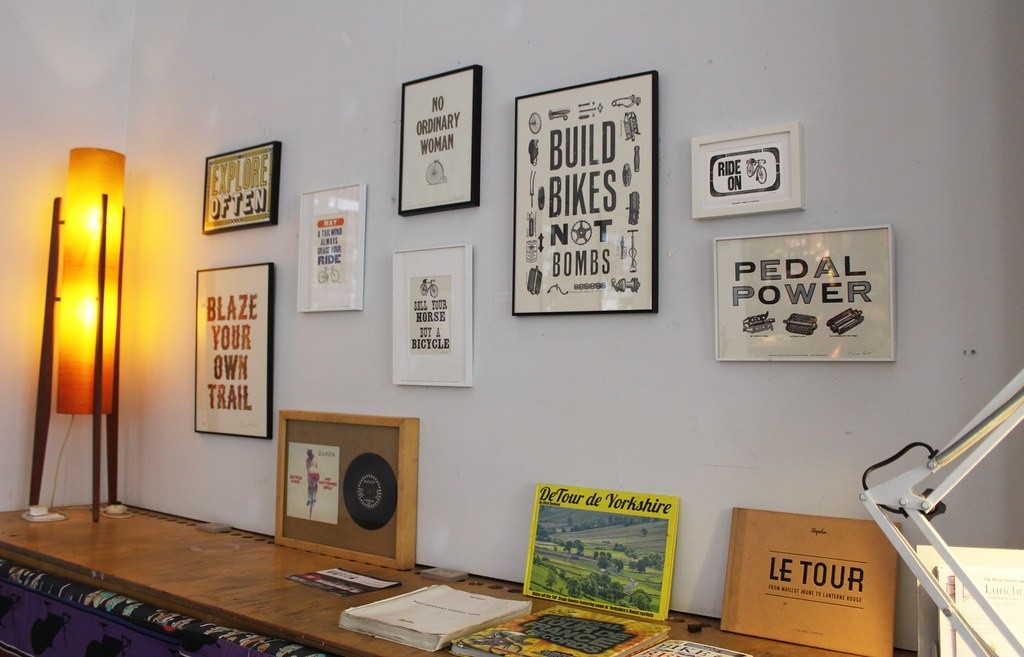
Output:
[29,148,127,523]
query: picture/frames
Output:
[297,183,367,313]
[195,263,275,440]
[274,410,420,569]
[393,245,472,388]
[512,70,659,316]
[714,225,897,360]
[397,65,482,217]
[202,142,281,234]
[692,122,804,220]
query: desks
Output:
[0,503,916,657]
